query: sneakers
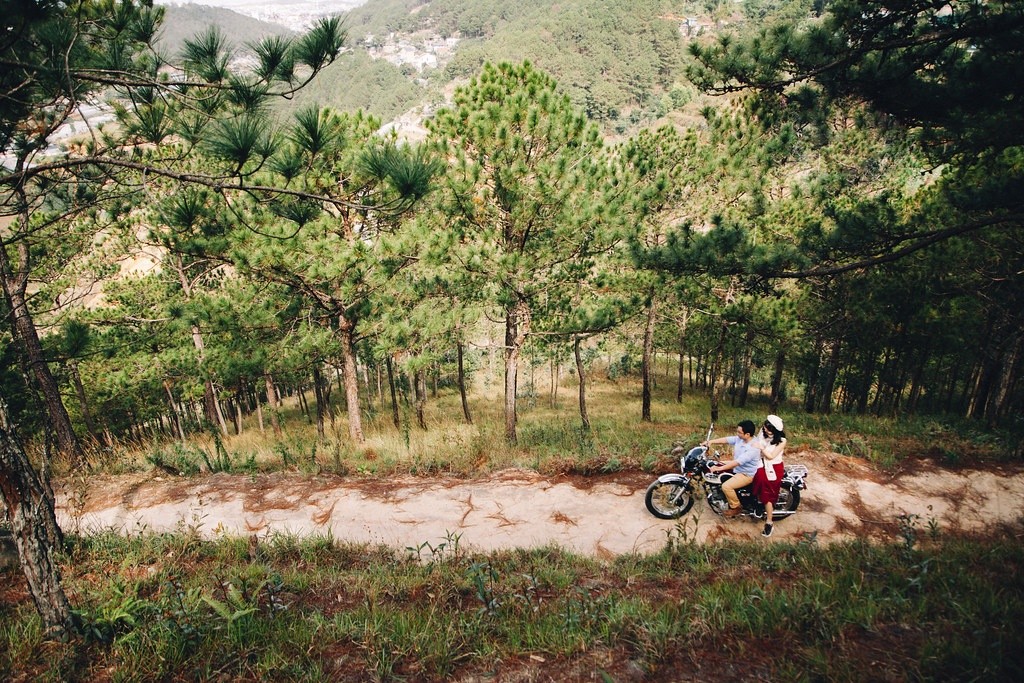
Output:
[754,502,763,518]
[761,521,774,538]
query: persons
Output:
[751,414,787,537]
[701,420,763,516]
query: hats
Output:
[767,415,783,431]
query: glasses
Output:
[738,430,742,434]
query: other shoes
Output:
[723,506,742,516]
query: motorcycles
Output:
[645,423,808,523]
